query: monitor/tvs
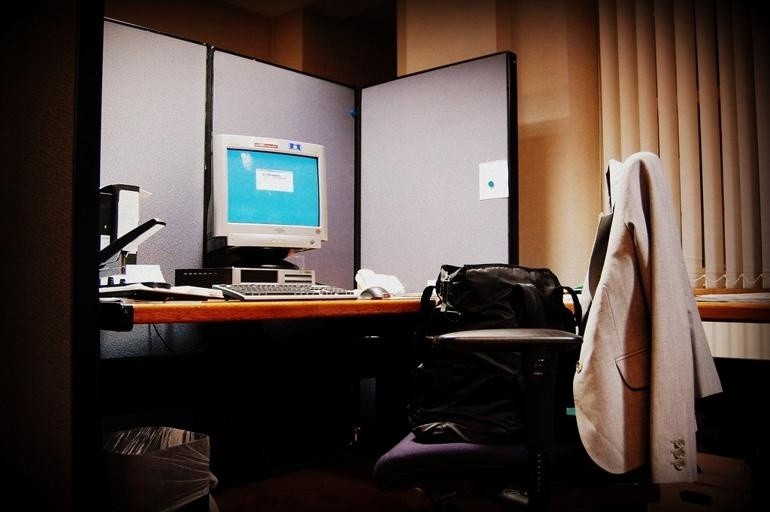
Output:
[207,134,328,270]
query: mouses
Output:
[361,286,390,299]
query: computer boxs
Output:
[175,267,316,289]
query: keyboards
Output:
[212,284,358,301]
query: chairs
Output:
[375,152,724,511]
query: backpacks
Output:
[406,263,583,442]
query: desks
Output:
[97,290,443,333]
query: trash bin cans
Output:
[110,426,209,511]
